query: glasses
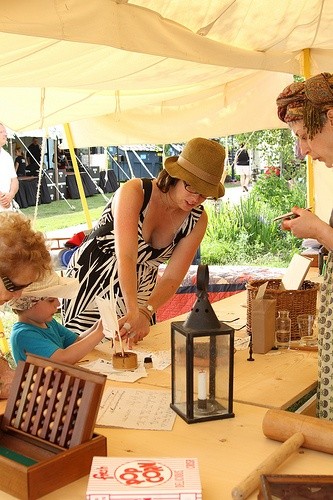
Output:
[183,180,215,200]
[1,273,33,292]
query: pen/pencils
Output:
[272,206,313,223]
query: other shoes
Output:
[242,184,248,192]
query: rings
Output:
[122,322,132,331]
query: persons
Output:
[26,137,43,176]
[0,121,26,216]
[14,146,27,177]
[0,211,51,400]
[60,137,226,344]
[6,268,104,369]
[275,71,333,421]
[234,141,252,192]
[51,144,69,172]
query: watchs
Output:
[143,302,156,315]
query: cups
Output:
[298,314,312,337]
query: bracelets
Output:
[139,307,152,320]
[139,311,152,321]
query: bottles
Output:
[275,310,291,349]
[318,245,328,275]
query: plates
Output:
[176,342,237,367]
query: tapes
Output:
[112,351,138,370]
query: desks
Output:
[80,284,322,418]
[0,373,333,500]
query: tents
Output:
[0,0,333,231]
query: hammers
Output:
[231,407,332,500]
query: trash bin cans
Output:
[90,165,100,194]
[57,168,66,200]
[45,170,55,201]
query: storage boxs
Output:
[245,279,319,340]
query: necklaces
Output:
[165,192,191,235]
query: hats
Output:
[164,137,225,201]
[276,72,333,141]
[22,269,80,299]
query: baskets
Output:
[245,278,321,340]
